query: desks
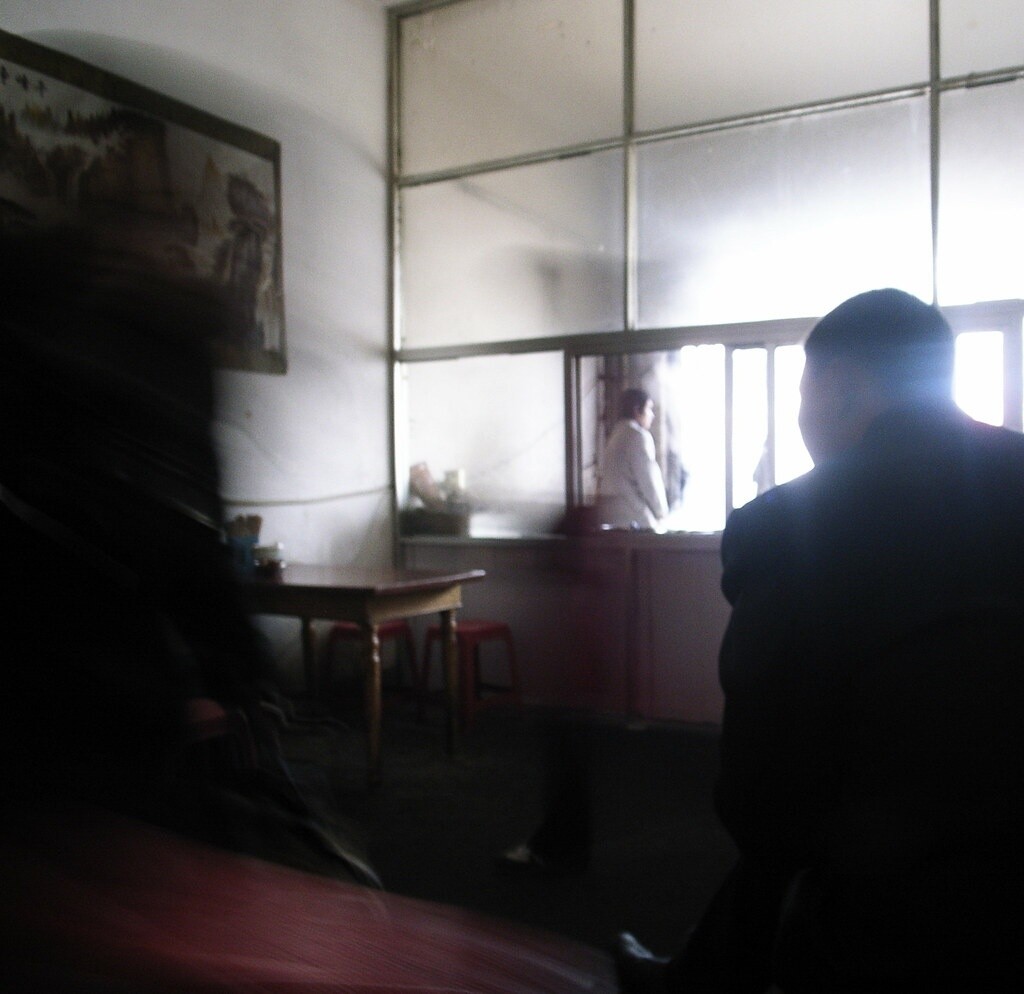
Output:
[240,565,487,790]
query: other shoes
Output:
[617,927,675,994]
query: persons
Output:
[621,289,1024,991]
[595,387,674,537]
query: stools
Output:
[320,616,529,729]
[168,693,261,798]
